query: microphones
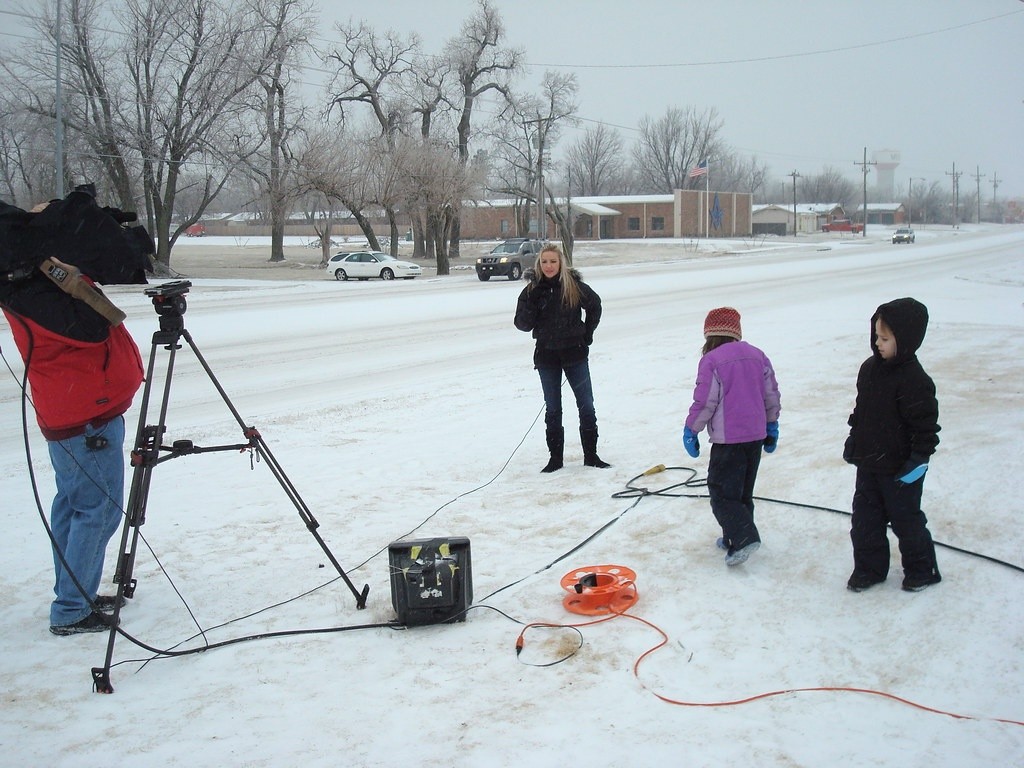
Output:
[122,212,137,223]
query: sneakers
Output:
[847,569,887,592]
[49,609,120,636]
[723,524,761,565]
[91,594,126,610]
[716,536,730,550]
[900,571,941,592]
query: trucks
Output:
[169,224,180,236]
[185,224,205,237]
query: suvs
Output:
[475,238,550,281]
[892,227,915,244]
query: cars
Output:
[327,251,422,281]
[822,220,863,234]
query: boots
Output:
[579,425,611,469]
[540,427,564,473]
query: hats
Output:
[704,306,742,342]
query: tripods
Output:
[93,281,370,695]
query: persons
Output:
[0,200,145,636]
[682,305,783,565]
[514,243,612,474]
[843,295,942,592]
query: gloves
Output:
[683,425,700,458]
[576,328,593,347]
[844,430,855,464]
[527,284,551,310]
[892,452,929,489]
[762,421,779,453]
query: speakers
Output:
[390,536,475,627]
